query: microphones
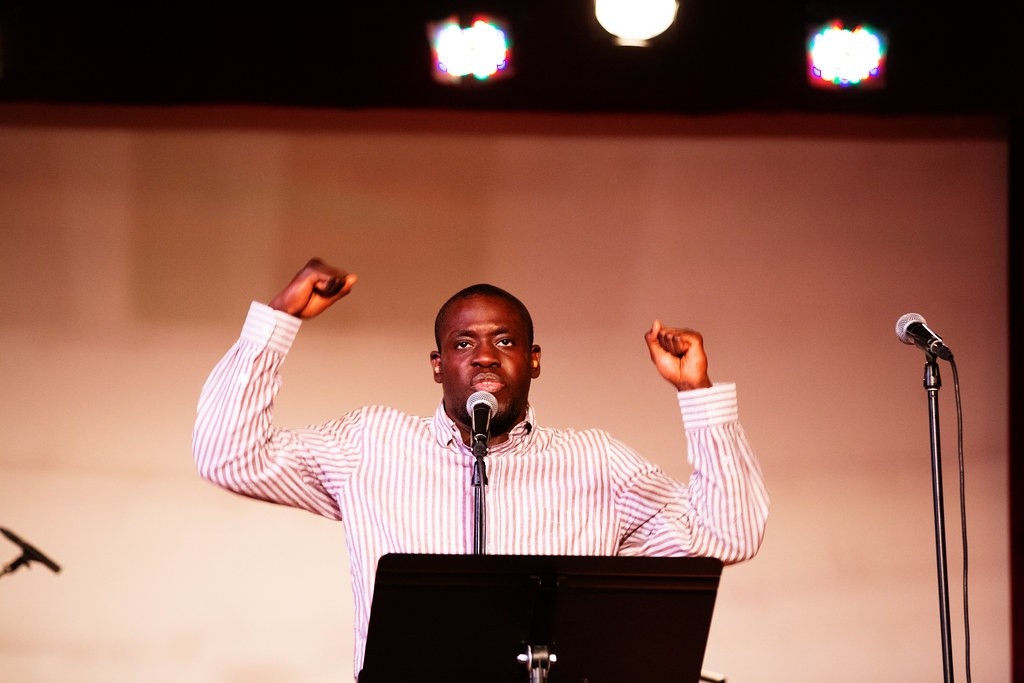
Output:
[0,527,60,573]
[895,313,954,362]
[467,390,499,487]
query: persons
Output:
[192,257,770,683]
